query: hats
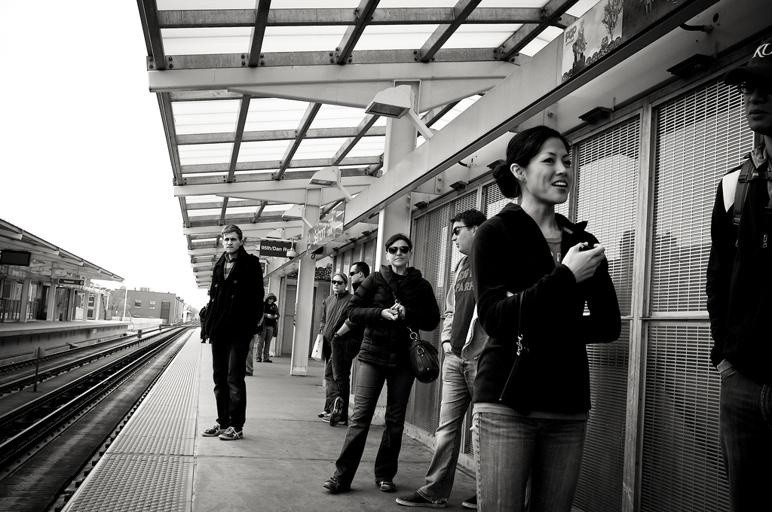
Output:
[724,39,772,84]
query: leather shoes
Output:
[256,358,261,362]
[263,359,273,363]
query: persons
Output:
[199,300,212,344]
[321,233,440,494]
[395,209,488,509]
[201,224,265,439]
[468,126,621,512]
[706,40,771,511]
[243,336,255,376]
[257,290,280,364]
[316,272,356,420]
[323,261,370,426]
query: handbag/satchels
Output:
[391,289,439,383]
[498,290,544,417]
[311,331,325,361]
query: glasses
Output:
[737,79,772,93]
[387,246,410,254]
[332,280,345,285]
[451,226,469,236]
[350,271,359,277]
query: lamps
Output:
[282,204,312,228]
[365,85,433,140]
[266,229,297,258]
[309,167,351,200]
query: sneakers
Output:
[330,397,344,426]
[318,411,327,417]
[219,427,243,440]
[462,495,477,509]
[376,478,394,491]
[324,477,351,492]
[323,416,345,424]
[396,491,446,508]
[202,424,226,436]
